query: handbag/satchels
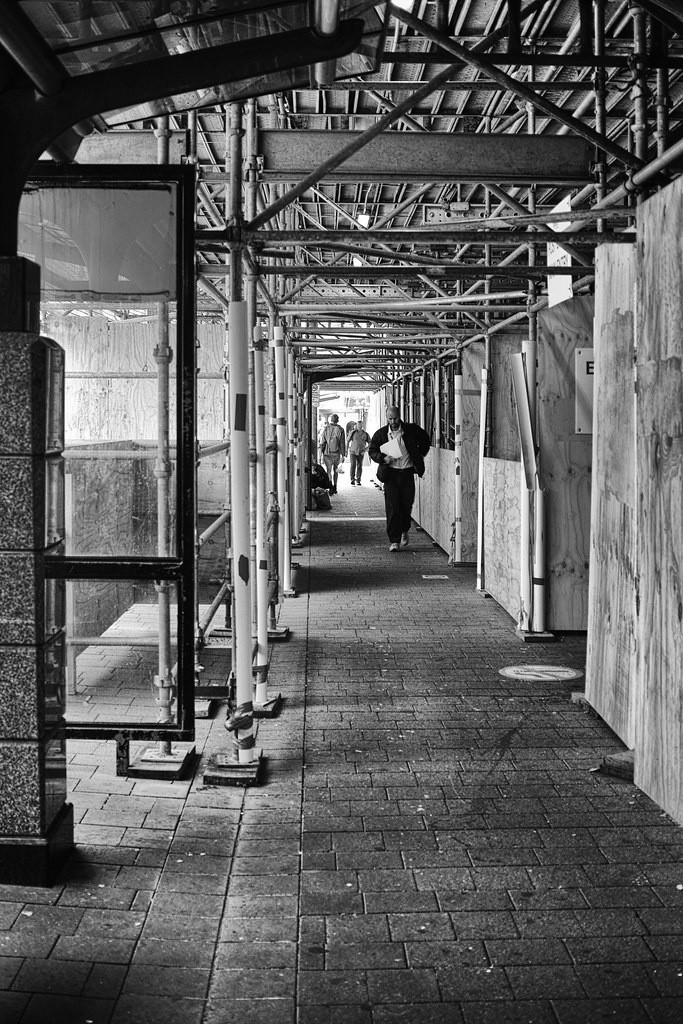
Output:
[313,488,332,509]
[335,462,345,474]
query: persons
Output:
[346,421,371,486]
[321,413,345,496]
[369,407,430,553]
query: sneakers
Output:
[388,542,400,552]
[401,531,409,547]
